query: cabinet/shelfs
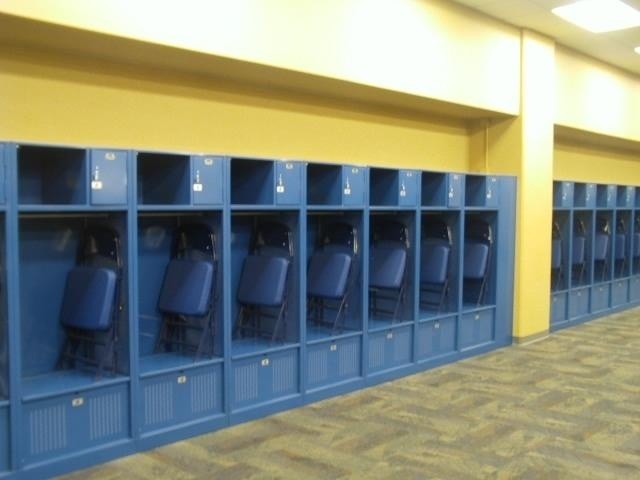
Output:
[2,144,135,479]
[369,156,512,388]
[126,150,229,447]
[549,180,639,332]
[303,161,369,405]
[228,155,305,428]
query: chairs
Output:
[233,223,295,343]
[305,222,360,336]
[156,217,221,360]
[55,222,124,382]
